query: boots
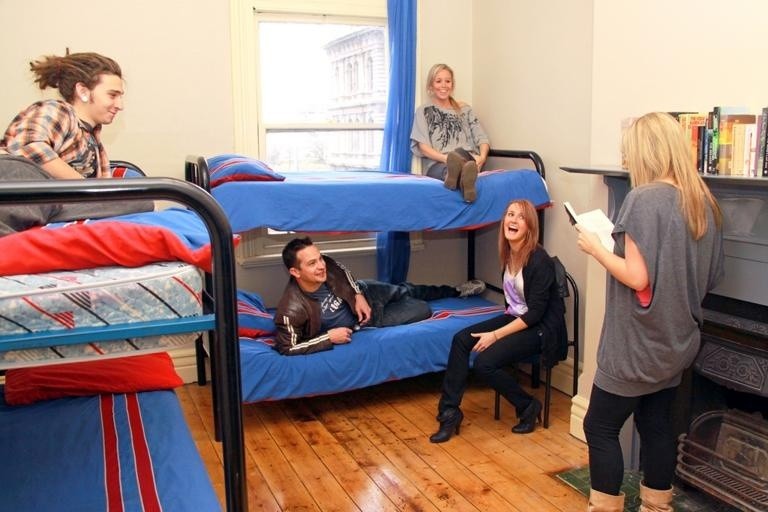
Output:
[588,488,625,512]
[639,481,674,512]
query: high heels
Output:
[430,406,463,443]
[512,397,542,433]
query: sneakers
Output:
[445,150,478,203]
[457,280,486,296]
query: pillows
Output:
[205,153,285,188]
[235,288,279,338]
[4,350,184,407]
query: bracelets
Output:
[492,331,498,341]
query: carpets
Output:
[546,463,740,512]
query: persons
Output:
[0,50,126,241]
[575,113,720,511]
[272,235,487,356]
[406,62,490,204]
[430,198,567,442]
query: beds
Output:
[1,160,248,512]
[184,150,546,443]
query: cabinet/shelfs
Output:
[559,166,768,512]
[495,271,579,428]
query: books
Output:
[563,199,616,254]
[619,107,768,180]
